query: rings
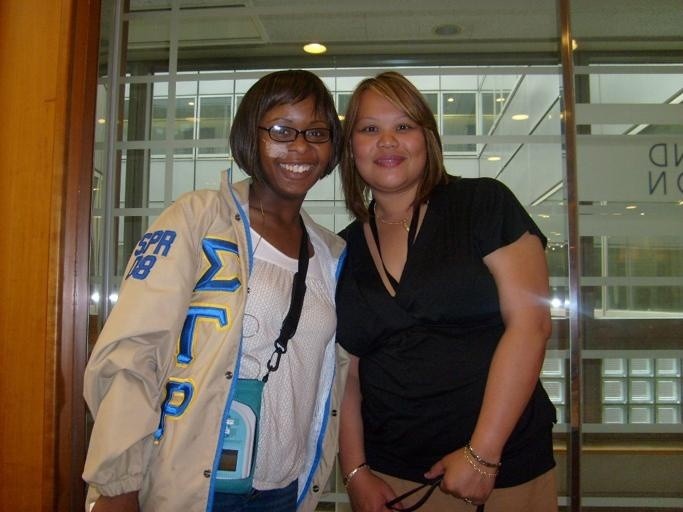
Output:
[464,499,474,507]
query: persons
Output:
[81,70,350,512]
[333,71,559,512]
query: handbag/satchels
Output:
[212,377,268,496]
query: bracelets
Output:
[463,442,501,480]
[343,463,371,487]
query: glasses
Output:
[257,125,333,145]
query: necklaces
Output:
[372,203,412,232]
